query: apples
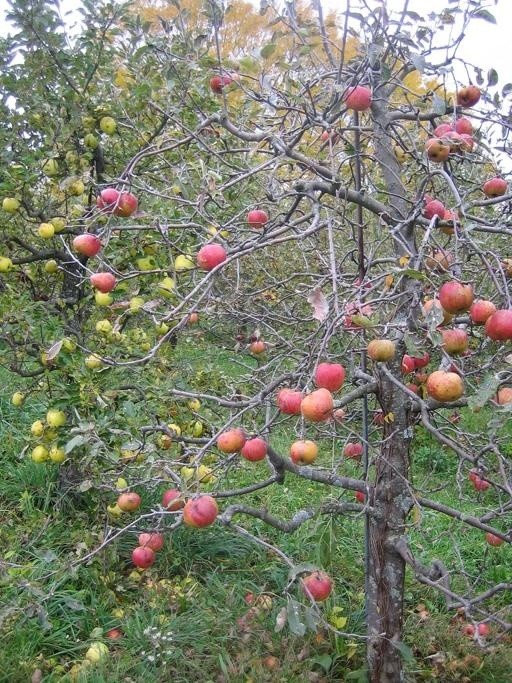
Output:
[132,547,155,568]
[139,131,153,146]
[173,185,181,195]
[463,625,474,640]
[41,159,69,178]
[66,180,85,196]
[279,388,310,414]
[487,533,502,546]
[168,423,181,438]
[78,155,88,169]
[354,280,372,292]
[96,321,110,334]
[180,421,188,432]
[188,398,200,412]
[3,197,19,212]
[65,151,77,169]
[332,409,345,424]
[132,234,156,258]
[434,124,455,139]
[424,299,454,326]
[345,303,370,331]
[45,260,58,273]
[218,430,245,452]
[97,189,119,214]
[31,420,44,436]
[394,141,410,162]
[442,329,467,354]
[156,321,169,333]
[118,493,140,511]
[193,465,211,483]
[207,224,229,240]
[115,477,128,488]
[501,259,512,278]
[414,351,429,366]
[129,297,145,310]
[401,354,416,372]
[300,388,333,422]
[474,479,488,490]
[342,86,371,109]
[424,196,445,221]
[441,211,457,236]
[484,310,511,340]
[198,245,226,271]
[62,336,77,352]
[158,278,177,299]
[181,466,196,485]
[478,624,489,636]
[442,131,460,151]
[408,384,417,392]
[47,409,67,429]
[242,439,268,460]
[251,340,264,354]
[459,86,480,106]
[140,342,150,354]
[321,127,338,145]
[455,118,474,136]
[368,340,394,361]
[74,235,100,256]
[468,300,495,322]
[107,504,123,519]
[175,255,194,276]
[416,372,425,384]
[113,332,129,347]
[68,203,86,220]
[439,282,473,314]
[158,435,172,450]
[302,573,331,601]
[49,445,67,462]
[115,192,138,217]
[85,352,103,368]
[187,420,203,437]
[51,216,67,232]
[374,409,384,426]
[483,178,508,197]
[495,388,512,405]
[465,654,480,668]
[0,257,13,272]
[470,468,482,481]
[84,134,97,151]
[356,490,364,501]
[84,195,97,207]
[461,350,472,358]
[445,360,458,372]
[107,630,127,639]
[211,67,239,93]
[317,364,344,392]
[184,496,218,527]
[162,489,184,511]
[291,439,318,466]
[427,370,464,402]
[12,392,24,405]
[32,445,49,463]
[136,256,156,270]
[99,116,115,134]
[208,476,218,485]
[418,611,430,620]
[460,134,473,155]
[91,272,116,293]
[245,595,272,615]
[138,533,163,551]
[264,656,279,670]
[130,328,147,344]
[81,118,96,133]
[424,137,450,162]
[248,211,267,228]
[427,250,452,272]
[41,352,59,365]
[39,223,54,238]
[86,641,109,661]
[451,660,465,670]
[95,290,115,306]
[186,313,199,326]
[345,443,362,458]
